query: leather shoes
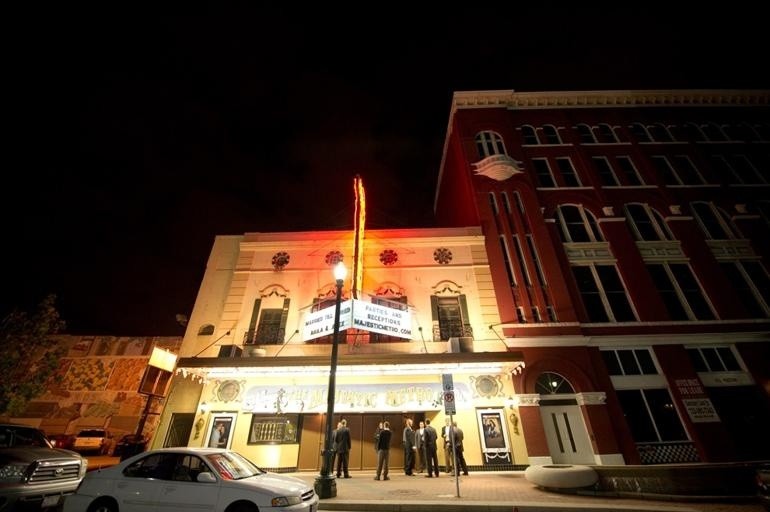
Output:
[407,468,467,479]
[374,476,391,480]
[336,473,351,478]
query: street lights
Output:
[312,260,349,500]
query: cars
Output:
[56,446,320,512]
[113,433,145,457]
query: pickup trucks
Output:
[71,426,116,454]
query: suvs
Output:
[0,421,90,511]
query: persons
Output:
[373,419,468,480]
[210,422,229,448]
[335,419,351,478]
[484,419,498,439]
[331,422,342,473]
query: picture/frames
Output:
[476,408,509,454]
[246,413,301,445]
[201,410,238,450]
